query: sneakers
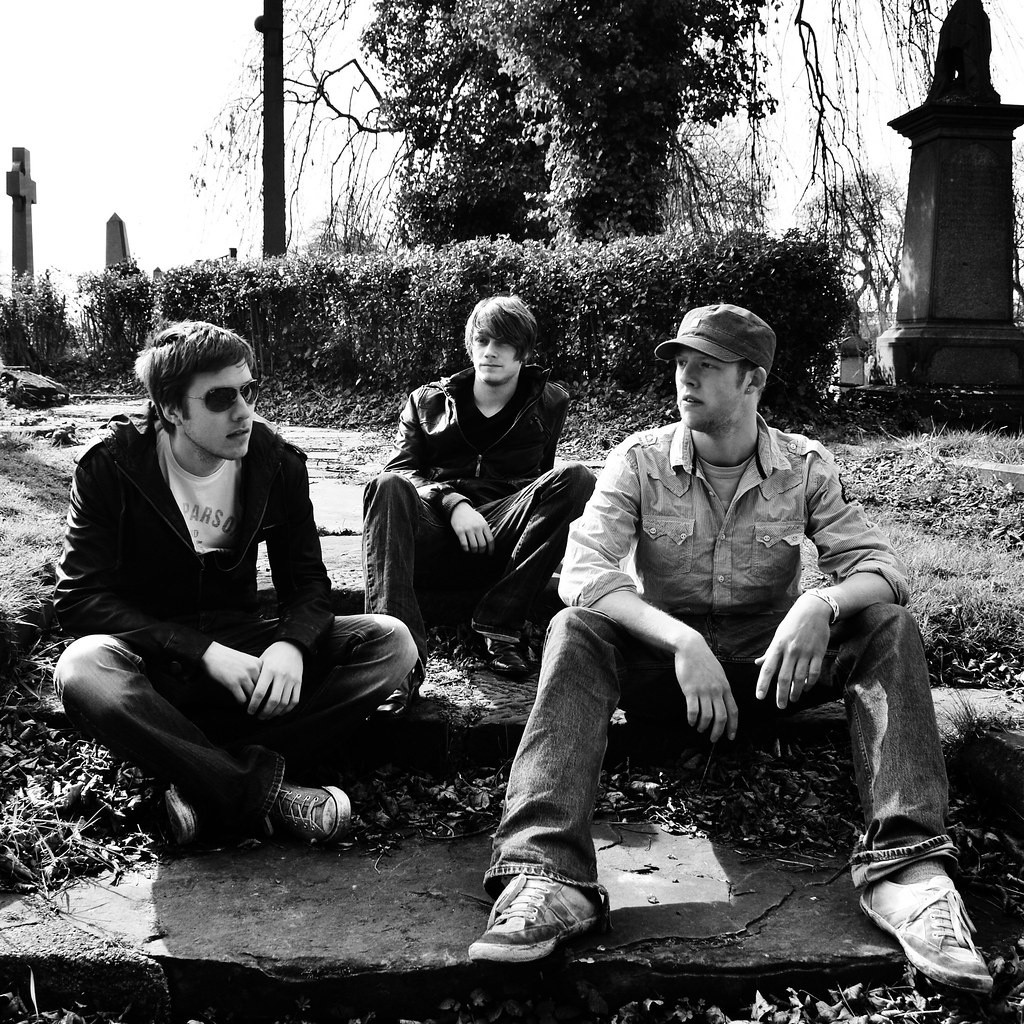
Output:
[165,783,203,844]
[274,780,351,840]
[469,873,600,962]
[859,874,995,993]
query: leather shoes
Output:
[371,685,418,721]
[482,635,528,673]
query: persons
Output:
[468,305,994,995]
[363,296,597,683]
[51,321,419,843]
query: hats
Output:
[656,304,777,374]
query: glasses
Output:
[183,378,259,412]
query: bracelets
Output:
[806,588,840,623]
